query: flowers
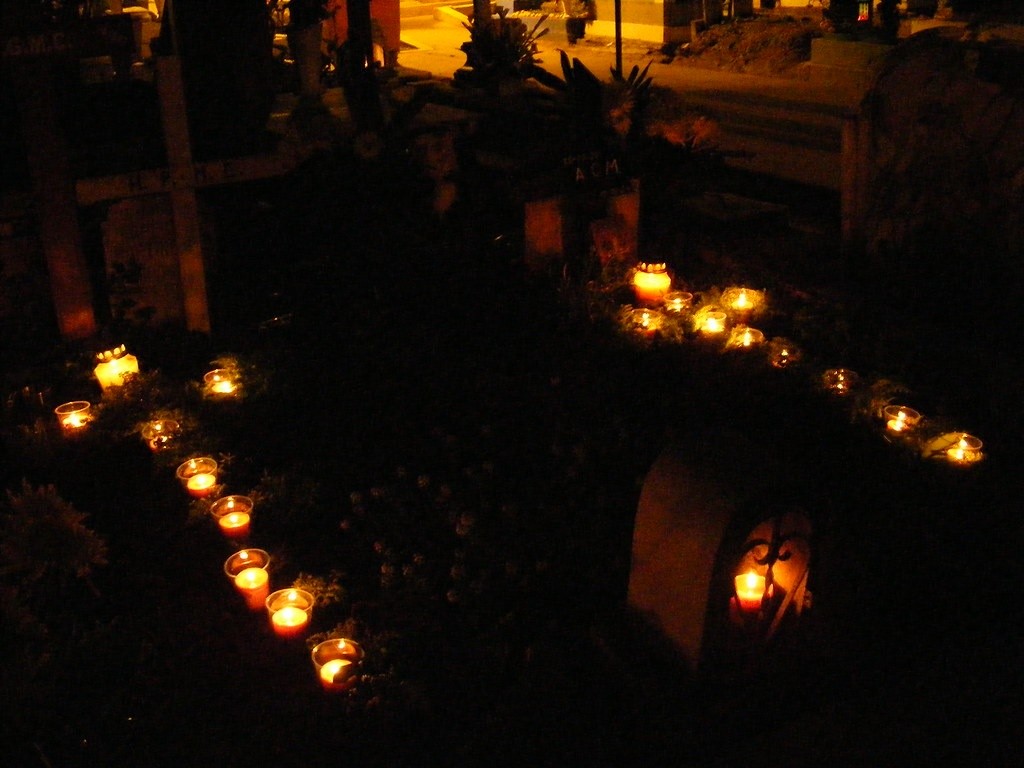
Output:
[0,1,1024,766]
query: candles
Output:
[735,572,774,613]
[882,405,920,438]
[204,369,232,395]
[635,262,671,301]
[143,419,181,451]
[663,291,692,312]
[177,457,217,497]
[224,549,269,608]
[265,588,315,642]
[942,434,982,464]
[699,312,726,331]
[732,327,764,347]
[209,494,253,542]
[55,401,91,436]
[311,638,365,695]
[725,288,763,323]
